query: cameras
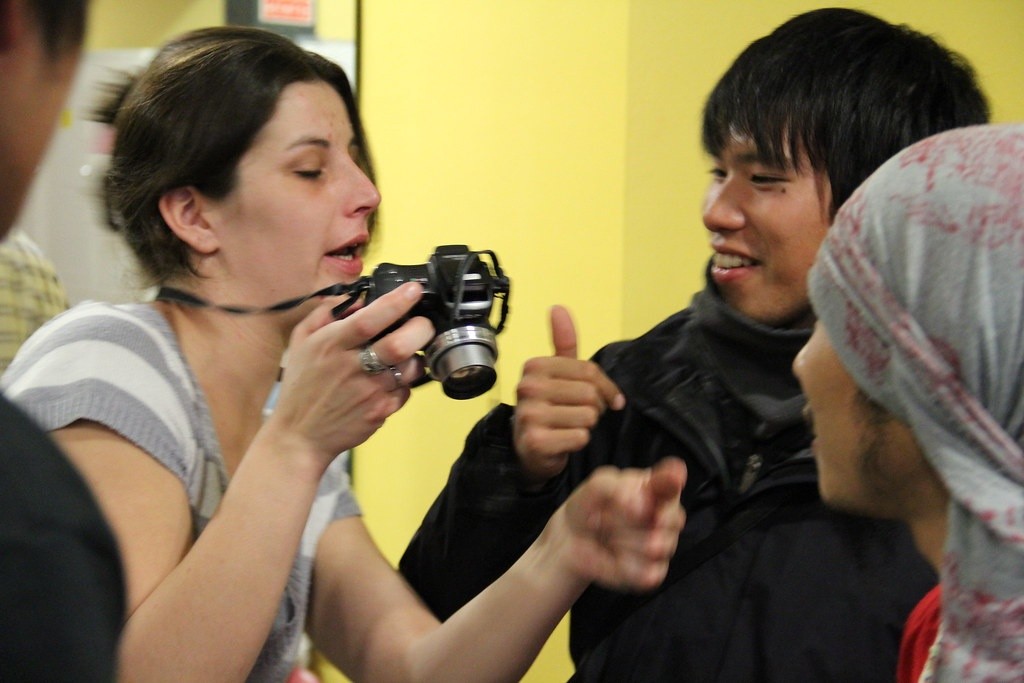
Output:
[362,244,510,400]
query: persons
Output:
[793,119,1024,683]
[0,27,683,683]
[395,6,989,683]
[0,0,125,682]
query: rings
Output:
[361,348,386,374]
[390,365,403,388]
